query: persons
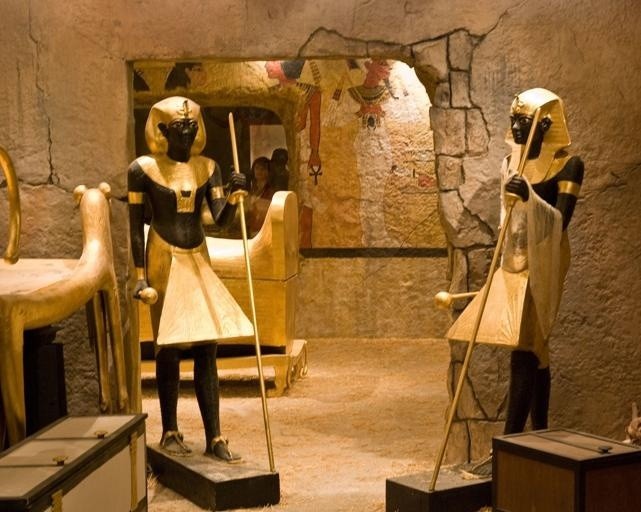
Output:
[469,112,586,476]
[325,57,409,130]
[249,155,281,200]
[256,61,324,250]
[125,117,253,461]
[269,148,291,193]
[163,63,209,90]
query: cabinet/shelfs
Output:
[493,427,640,511]
[0,415,147,512]
